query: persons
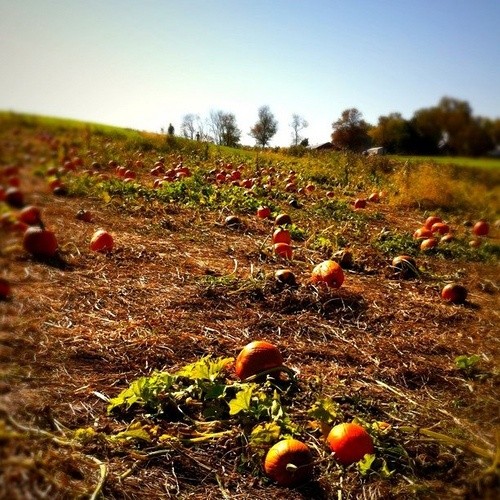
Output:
[167,122,175,135]
[193,130,201,141]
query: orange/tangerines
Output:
[0,129,489,305]
[326,422,375,464]
[235,340,284,382]
[264,439,315,484]
